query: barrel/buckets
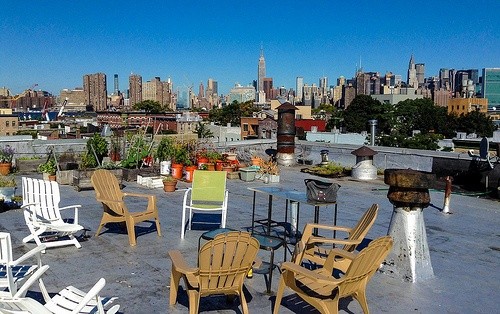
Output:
[160,161,171,175]
[172,163,183,179]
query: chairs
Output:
[16,177,85,254]
[169,231,260,314]
[273,203,394,314]
[91,169,162,246]
[0,232,120,314]
[180,170,229,240]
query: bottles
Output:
[247,268,253,278]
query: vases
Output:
[0,163,12,175]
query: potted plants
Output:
[162,176,178,192]
[39,159,57,181]
[110,134,121,161]
[157,136,280,183]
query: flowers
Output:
[0,146,15,163]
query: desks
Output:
[198,228,286,293]
[247,186,342,248]
